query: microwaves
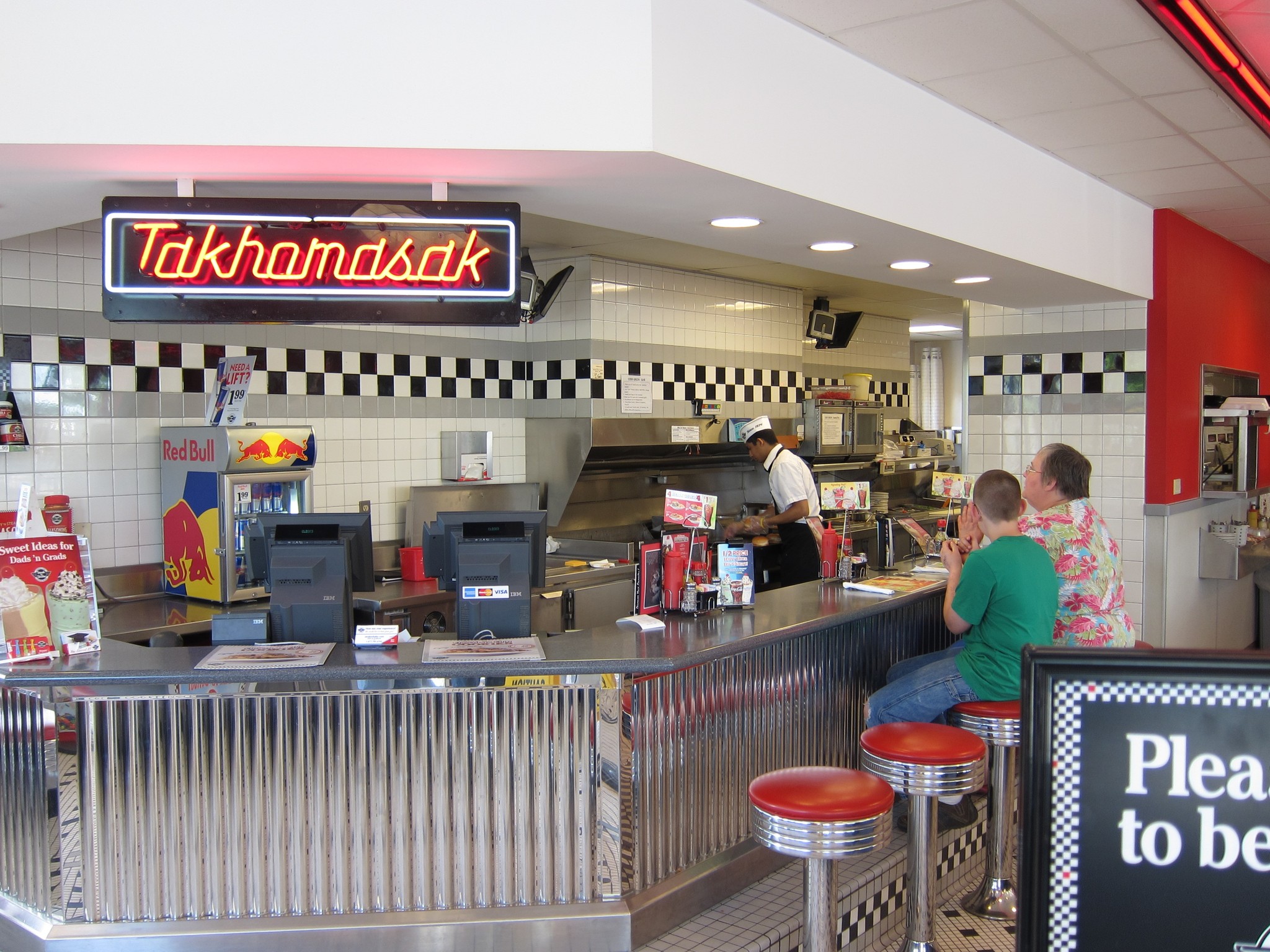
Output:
[797,398,884,464]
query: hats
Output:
[739,416,772,443]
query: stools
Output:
[954,699,1022,922]
[858,723,994,952]
[746,763,896,952]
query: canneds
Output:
[0,401,25,447]
[41,494,73,533]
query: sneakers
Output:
[898,794,978,833]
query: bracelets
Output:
[759,516,768,530]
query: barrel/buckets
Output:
[842,373,873,401]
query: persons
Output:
[956,442,1137,650]
[861,469,1062,834]
[723,415,826,588]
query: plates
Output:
[869,492,890,514]
[668,503,702,524]
[1211,532,1235,547]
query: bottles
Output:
[661,537,722,613]
[821,521,868,580]
[1247,505,1258,530]
[932,519,949,554]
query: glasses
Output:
[1025,463,1042,473]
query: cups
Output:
[910,347,944,431]
[722,578,753,604]
[933,477,973,498]
[704,504,714,526]
[824,488,867,510]
[1,583,53,652]
[46,581,90,657]
